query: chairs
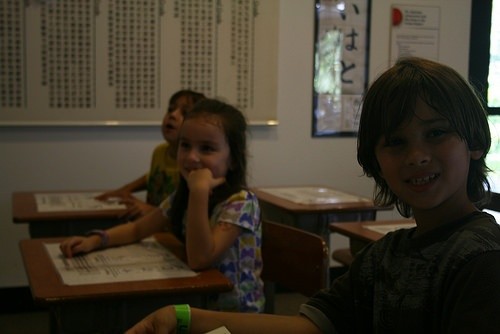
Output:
[332,249,354,268]
[260,218,330,314]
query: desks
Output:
[331,219,416,244]
[11,188,138,238]
[18,239,235,314]
[254,186,394,258]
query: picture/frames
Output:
[310,0,371,138]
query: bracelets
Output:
[87,229,107,250]
[174,303,191,334]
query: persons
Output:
[93,90,207,259]
[57,98,267,314]
[124,57,500,334]
